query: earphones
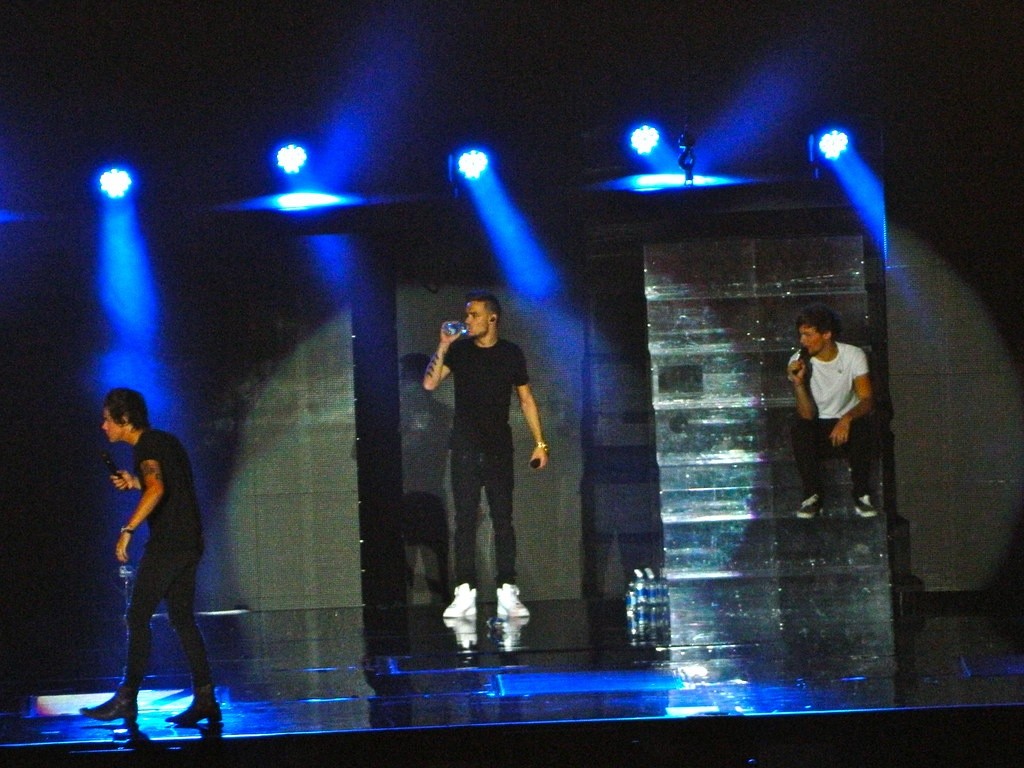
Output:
[489,317,495,322]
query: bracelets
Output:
[535,441,547,452]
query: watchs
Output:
[120,526,135,535]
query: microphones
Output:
[530,458,540,469]
[792,346,808,375]
[101,451,130,491]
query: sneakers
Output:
[444,618,478,650]
[487,617,529,652]
[442,582,477,619]
[496,583,530,618]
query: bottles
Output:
[625,568,670,609]
[444,321,473,337]
[119,563,134,603]
[628,604,669,633]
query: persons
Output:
[787,305,880,519]
[79,388,222,723]
[424,293,547,618]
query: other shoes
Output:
[797,494,822,518]
[855,496,878,517]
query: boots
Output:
[166,684,223,725]
[80,685,138,723]
